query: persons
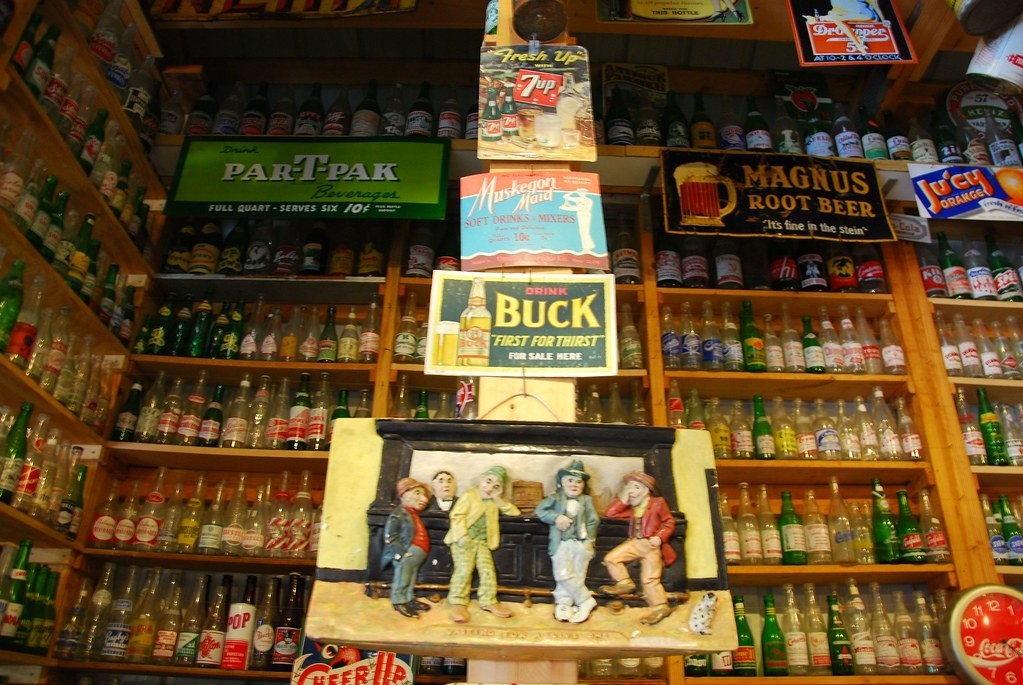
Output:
[378,458,677,625]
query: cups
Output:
[516,107,543,141]
[534,113,561,147]
[432,319,460,366]
[561,129,581,149]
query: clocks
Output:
[939,584,1023,685]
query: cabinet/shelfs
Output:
[0,0,1023,685]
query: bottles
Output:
[576,61,1023,674]
[555,72,585,130]
[0,3,477,674]
[457,277,493,369]
[481,89,502,140]
[500,86,518,135]
